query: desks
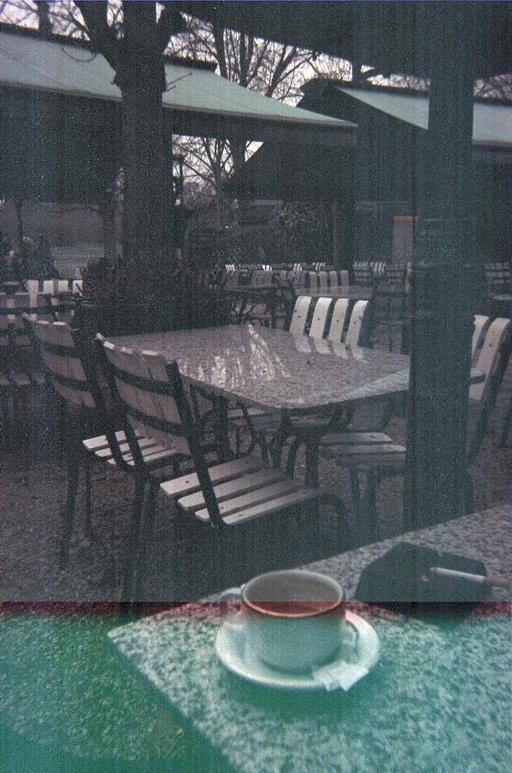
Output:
[101,322,484,544]
[107,505,512,773]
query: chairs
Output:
[20,308,222,599]
[202,293,375,516]
[305,312,509,544]
[1,256,510,459]
[92,331,350,628]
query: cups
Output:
[218,569,346,675]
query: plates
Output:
[214,606,382,690]
